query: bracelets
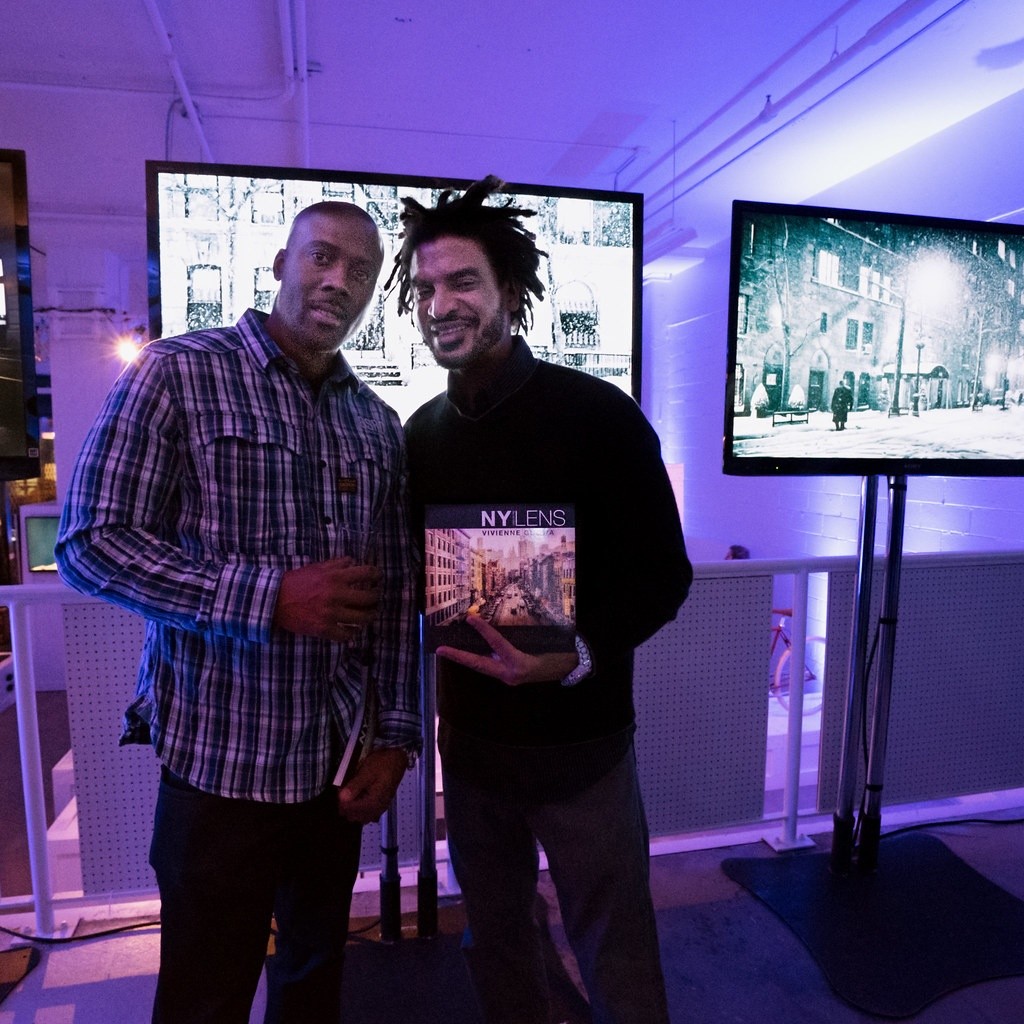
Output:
[562,635,593,688]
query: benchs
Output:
[766,409,809,427]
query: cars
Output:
[989,387,1004,406]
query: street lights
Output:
[913,343,924,415]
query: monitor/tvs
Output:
[145,160,644,428]
[722,200,1024,479]
[0,149,42,484]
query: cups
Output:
[331,522,382,647]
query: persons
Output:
[725,545,750,560]
[384,173,693,1024]
[831,381,853,431]
[53,203,425,1024]
